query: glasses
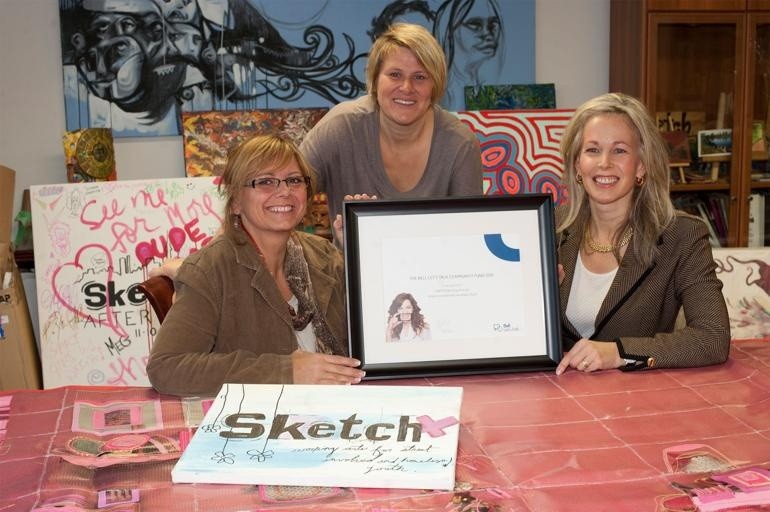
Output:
[240,175,311,193]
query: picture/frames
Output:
[341,194,563,382]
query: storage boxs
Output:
[1,164,42,392]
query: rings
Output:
[580,359,589,368]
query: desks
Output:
[1,337,768,507]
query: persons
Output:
[385,291,431,341]
[144,133,380,398]
[547,92,730,374]
[146,24,484,309]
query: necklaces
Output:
[585,223,633,254]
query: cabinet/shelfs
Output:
[608,2,769,245]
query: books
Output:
[673,190,766,248]
